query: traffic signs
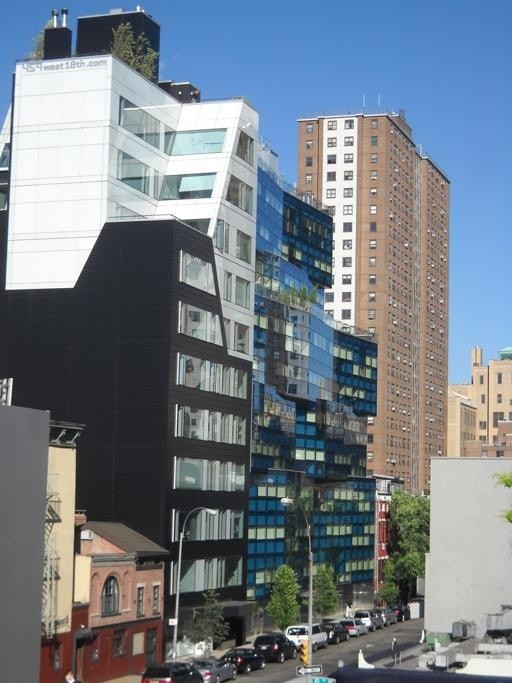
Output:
[295,665,323,675]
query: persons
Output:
[344,599,350,617]
[63,667,81,682]
[419,627,427,644]
[391,635,400,658]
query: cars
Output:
[193,658,237,683]
[339,619,368,637]
[222,648,266,674]
[323,622,350,644]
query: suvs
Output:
[255,634,296,662]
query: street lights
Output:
[171,506,216,659]
[281,496,314,683]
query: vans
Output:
[375,608,409,626]
[354,610,384,632]
[141,662,203,683]
[285,624,329,652]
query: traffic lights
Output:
[300,640,308,663]
